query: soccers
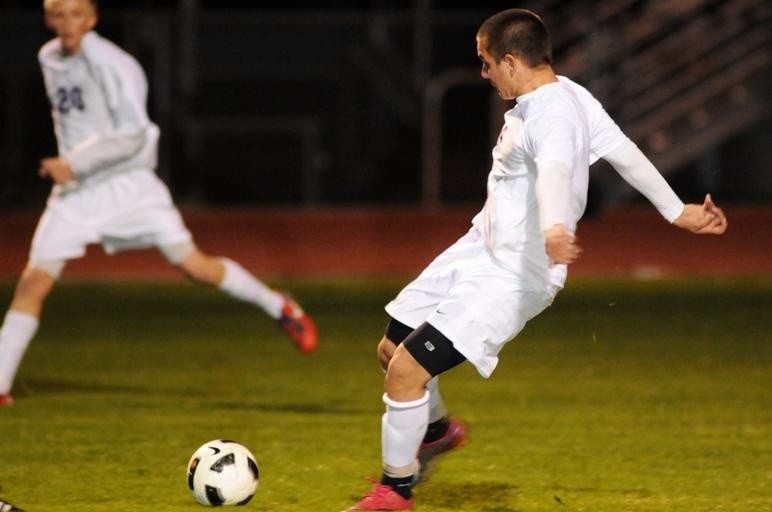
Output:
[186,439,259,506]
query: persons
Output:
[1,1,319,403]
[343,8,727,511]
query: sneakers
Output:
[275,292,320,355]
[342,476,415,512]
[416,414,468,472]
